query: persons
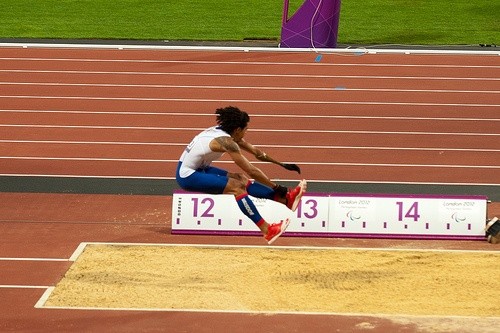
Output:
[176,106,307,244]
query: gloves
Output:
[284,163,300,174]
[272,184,288,198]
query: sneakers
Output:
[264,218,290,245]
[285,178,307,212]
